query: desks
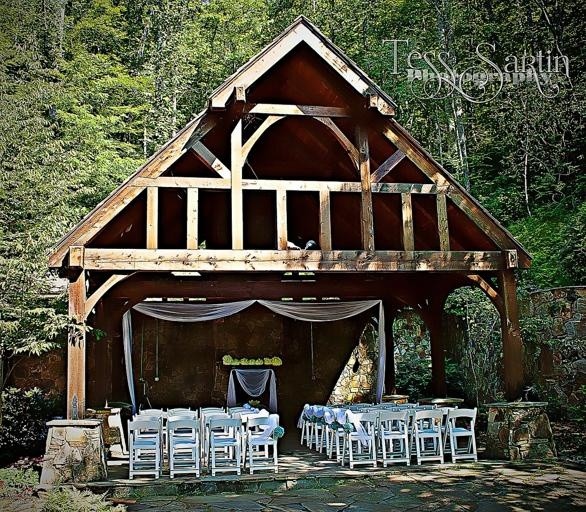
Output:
[86,406,126,457]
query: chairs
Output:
[127,405,285,482]
[295,400,481,470]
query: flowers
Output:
[221,354,283,367]
